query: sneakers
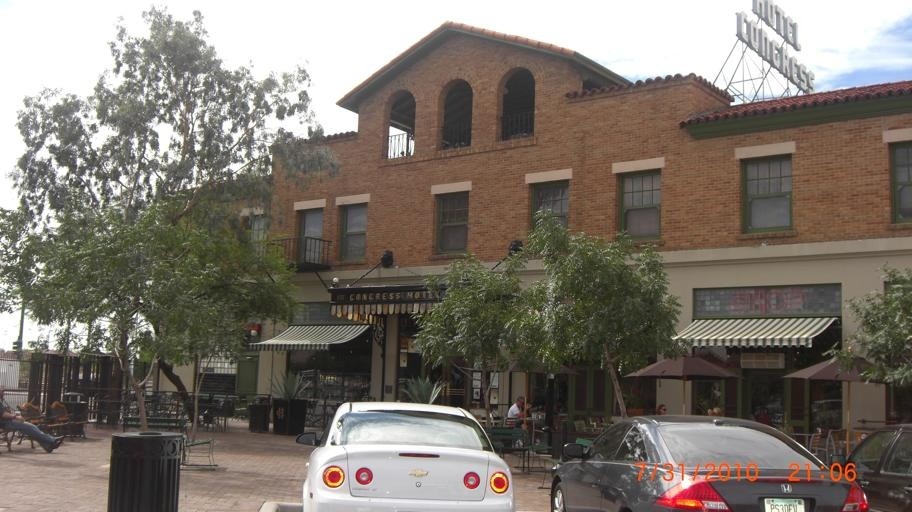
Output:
[44,436,65,453]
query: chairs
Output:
[808,427,847,465]
[0,400,88,451]
[488,413,613,489]
[180,426,217,467]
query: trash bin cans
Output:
[248,397,270,433]
[61,392,89,438]
[108,431,184,512]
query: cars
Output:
[549,414,869,512]
[830,423,911,512]
[302,402,513,512]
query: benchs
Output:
[118,400,187,433]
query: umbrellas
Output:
[471,346,582,431]
[619,348,739,416]
[782,346,892,456]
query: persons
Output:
[507,395,532,429]
[707,407,721,416]
[657,404,668,414]
[0,386,65,453]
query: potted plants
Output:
[263,369,313,435]
